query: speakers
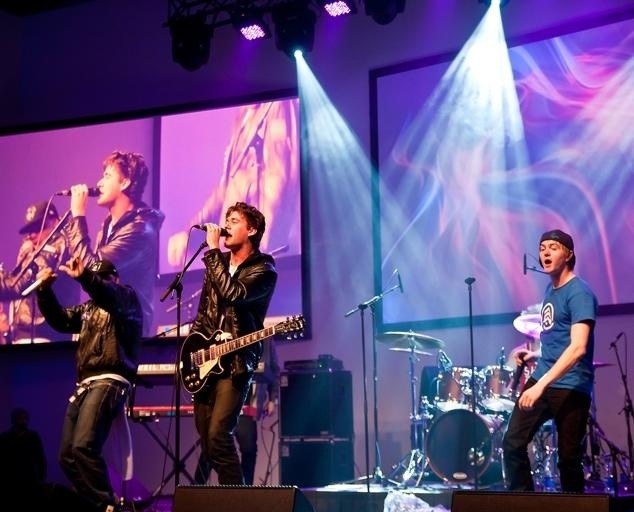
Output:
[279,369,353,437]
[278,440,353,486]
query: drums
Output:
[526,434,548,477]
[480,365,517,412]
[426,408,502,486]
[436,367,479,411]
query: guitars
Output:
[179,313,306,393]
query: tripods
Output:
[328,308,402,487]
[385,346,436,488]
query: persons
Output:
[58,148,166,338]
[187,202,279,486]
[34,254,144,511]
[232,326,283,486]
[504,229,599,493]
[0,408,48,511]
[0,200,82,344]
[506,303,544,405]
[166,99,301,270]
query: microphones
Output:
[10,245,35,278]
[56,186,100,197]
[512,353,527,390]
[398,273,404,293]
[21,270,54,297]
[200,224,227,236]
[523,254,527,275]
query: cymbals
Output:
[388,349,432,357]
[594,362,616,368]
[513,314,541,339]
[375,332,444,348]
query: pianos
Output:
[131,405,256,419]
[137,361,274,386]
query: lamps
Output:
[364,0,408,27]
[321,1,355,17]
[273,0,318,60]
[167,0,213,68]
[231,8,269,45]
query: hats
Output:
[18,201,59,234]
[539,230,576,271]
[88,260,118,273]
